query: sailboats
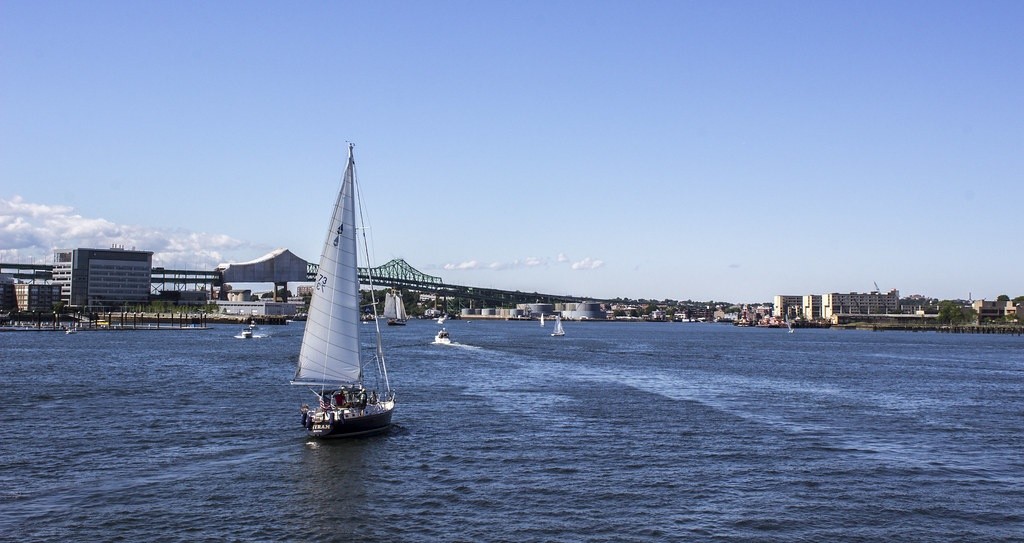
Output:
[539,312,545,327]
[383,291,409,326]
[289,144,396,442]
[550,313,566,336]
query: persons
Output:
[319,388,377,407]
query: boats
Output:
[67,331,77,334]
[242,328,252,340]
[435,330,452,344]
